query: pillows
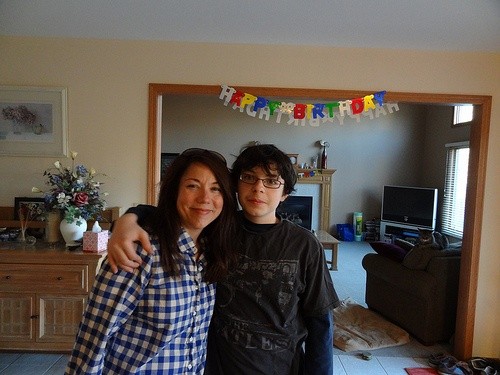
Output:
[392,238,415,252]
[367,240,407,264]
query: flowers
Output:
[25,151,112,226]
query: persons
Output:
[104,144,341,375]
[62,146,243,375]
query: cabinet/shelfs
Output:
[294,165,337,233]
[0,241,108,355]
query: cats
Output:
[414,228,449,249]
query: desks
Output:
[309,228,341,272]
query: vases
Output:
[59,214,88,248]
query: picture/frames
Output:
[0,84,70,160]
[13,196,48,240]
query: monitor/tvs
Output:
[381,185,438,230]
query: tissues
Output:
[82,220,110,254]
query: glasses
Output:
[239,173,285,189]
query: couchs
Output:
[361,240,464,347]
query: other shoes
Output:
[428,352,498,375]
